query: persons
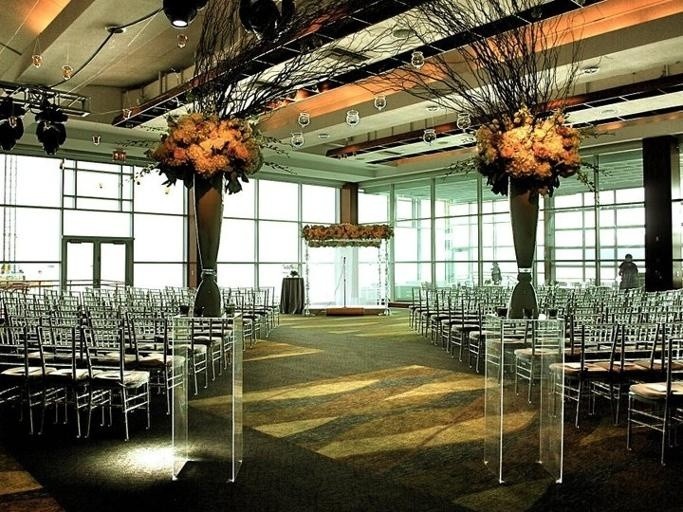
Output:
[491,261,502,285]
[618,253,640,289]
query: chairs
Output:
[409,283,682,468]
[1,282,280,440]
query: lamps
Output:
[0,84,69,157]
[161,0,281,43]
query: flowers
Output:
[428,104,613,198]
[113,106,298,199]
[298,221,393,249]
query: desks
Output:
[278,277,304,316]
